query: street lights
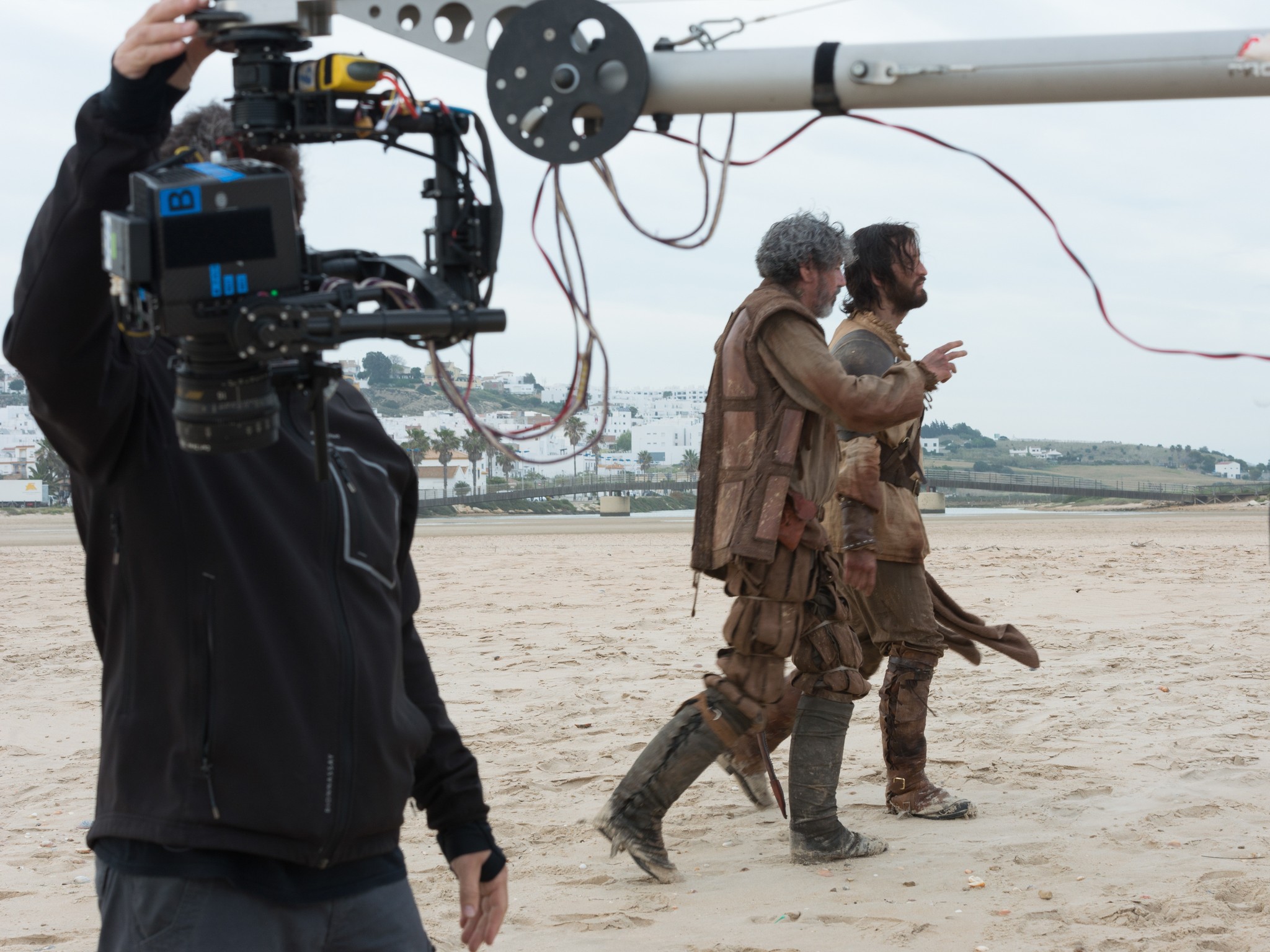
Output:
[680,463,689,472]
[406,448,420,465]
[516,450,530,490]
[578,453,660,485]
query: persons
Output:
[594,205,966,882]
[713,222,973,821]
[3,3,509,952]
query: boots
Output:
[719,676,804,808]
[593,685,728,883]
[789,693,889,866]
[878,646,976,818]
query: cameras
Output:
[99,25,514,485]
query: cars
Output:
[48,495,59,507]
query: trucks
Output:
[0,479,42,508]
[27,484,49,507]
